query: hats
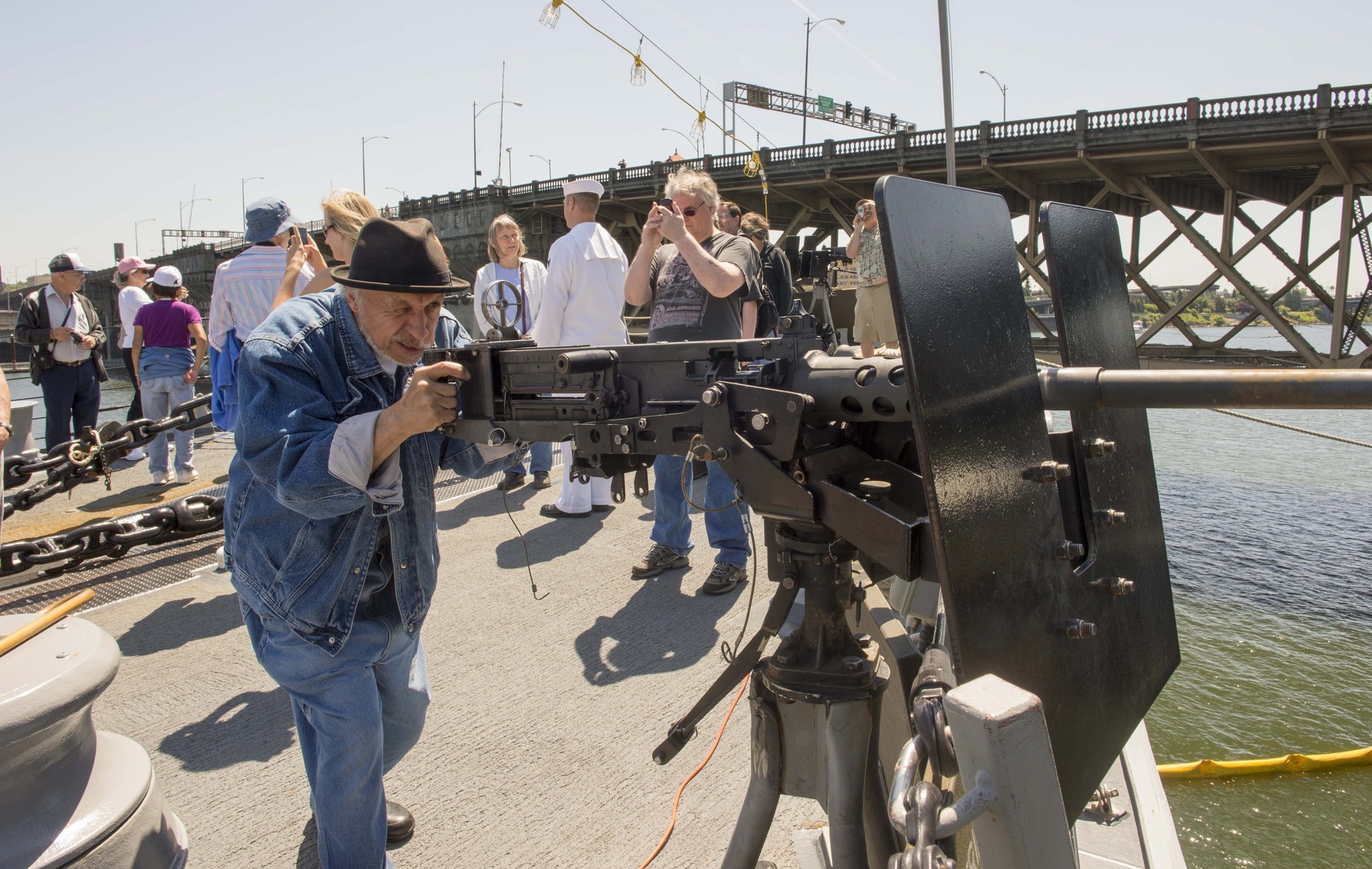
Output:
[48,253,95,273]
[562,179,604,199]
[146,265,182,287]
[856,199,875,211]
[329,216,470,293]
[243,196,299,242]
[118,256,156,274]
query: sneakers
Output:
[703,561,748,595]
[632,543,690,578]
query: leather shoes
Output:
[385,798,415,842]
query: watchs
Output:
[0,422,14,437]
[94,336,100,347]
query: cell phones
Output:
[298,227,309,246]
[660,198,674,214]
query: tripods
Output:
[809,285,839,351]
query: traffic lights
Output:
[891,113,897,130]
[846,101,853,119]
[864,106,871,124]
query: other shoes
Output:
[142,444,175,453]
[592,505,609,512]
[177,469,199,484]
[153,470,175,485]
[533,470,552,489]
[121,449,146,461]
[539,504,592,518]
[497,471,525,489]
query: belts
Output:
[56,356,93,367]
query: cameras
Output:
[70,332,83,345]
[858,205,865,218]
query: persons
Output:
[131,266,208,485]
[207,195,314,355]
[0,367,14,548]
[13,253,113,477]
[111,256,175,461]
[271,188,381,312]
[472,166,900,594]
[224,220,534,869]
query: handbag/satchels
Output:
[117,324,127,350]
[29,349,57,386]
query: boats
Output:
[1133,320,1146,334]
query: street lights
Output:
[530,154,551,179]
[803,17,845,157]
[242,177,265,244]
[135,219,156,257]
[15,264,29,289]
[7,278,9,311]
[180,198,212,249]
[980,70,1005,137]
[386,187,405,197]
[662,128,699,166]
[474,101,523,187]
[35,257,50,285]
[363,136,389,196]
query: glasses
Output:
[680,201,705,217]
[718,214,735,220]
[136,270,150,274]
[64,271,86,278]
[322,224,334,237]
